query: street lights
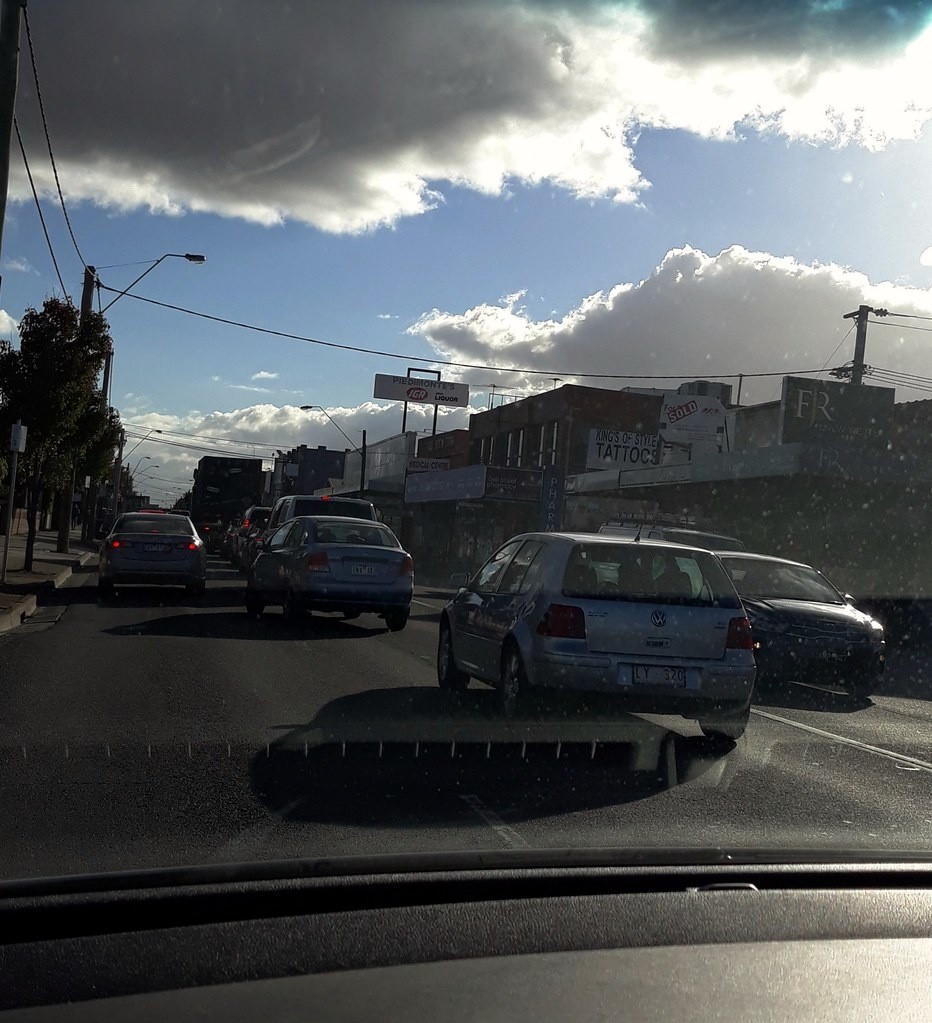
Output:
[55,254,208,553]
[300,405,366,499]
[112,430,162,518]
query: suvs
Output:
[584,518,749,603]
[250,494,383,573]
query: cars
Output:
[166,505,273,572]
[436,530,759,744]
[97,511,212,605]
[245,514,415,632]
[706,548,887,702]
